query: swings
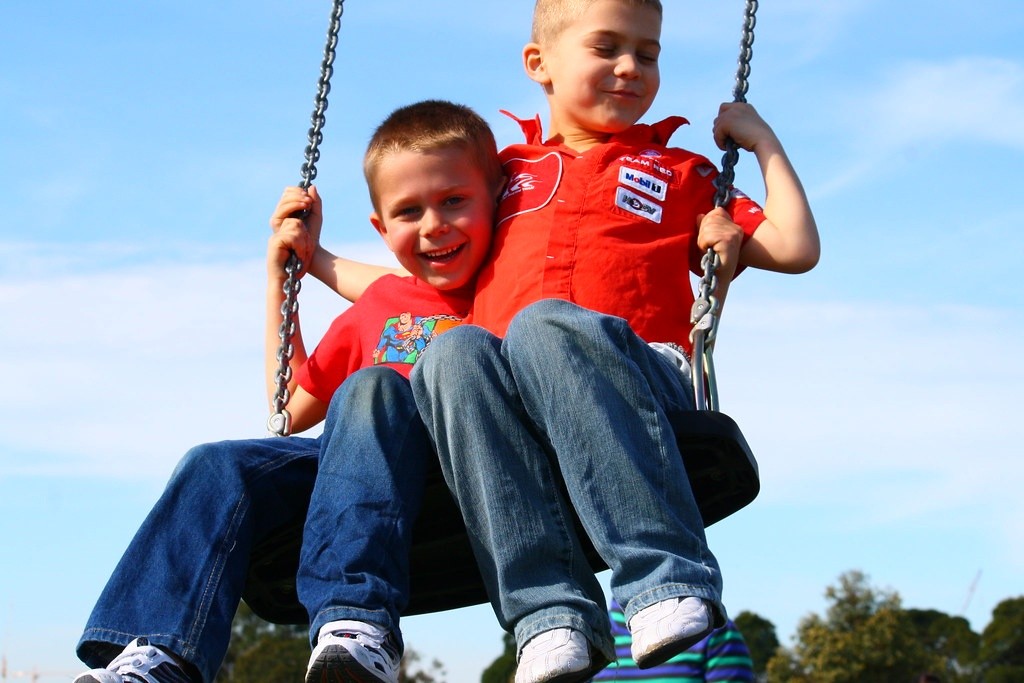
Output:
[240,0,766,625]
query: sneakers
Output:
[629,596,714,670]
[304,620,400,683]
[72,637,194,683]
[514,627,595,683]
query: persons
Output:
[269,0,820,682]
[70,100,508,683]
[588,590,753,683]
